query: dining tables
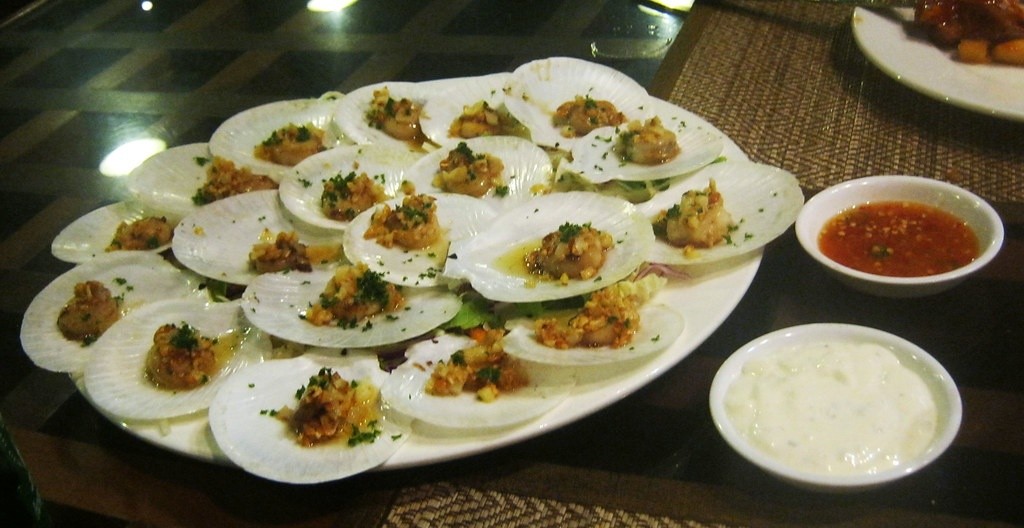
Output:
[0,1,1024,528]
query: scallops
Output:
[18,55,806,485]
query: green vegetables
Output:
[114,97,752,446]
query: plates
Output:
[852,0,1024,121]
[62,121,766,471]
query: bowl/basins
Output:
[709,323,963,494]
[795,175,1004,300]
